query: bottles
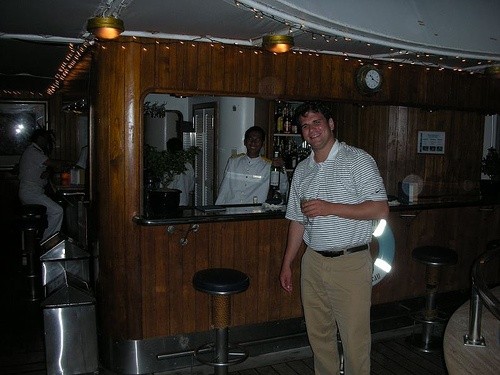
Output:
[268,137,313,204]
[275,100,300,134]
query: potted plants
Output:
[143,144,202,215]
[481,147,500,202]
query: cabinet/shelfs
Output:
[274,102,314,172]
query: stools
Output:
[405,245,458,353]
[192,268,250,375]
[17,212,46,301]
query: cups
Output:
[301,198,320,213]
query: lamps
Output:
[87,15,125,40]
[263,35,295,53]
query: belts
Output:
[313,243,368,257]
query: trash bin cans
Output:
[38,231,99,375]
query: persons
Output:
[19,129,70,243]
[215,126,289,206]
[157,138,194,206]
[280,102,389,375]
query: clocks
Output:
[356,64,385,93]
